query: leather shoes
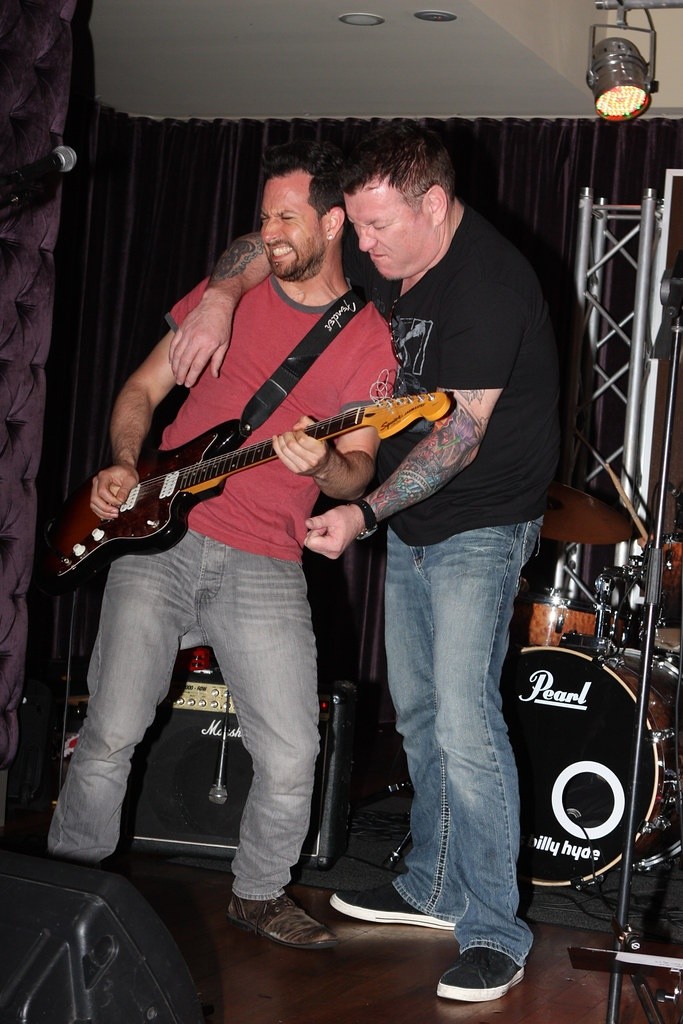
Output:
[226,890,340,949]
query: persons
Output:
[169,121,562,1003]
[46,139,400,951]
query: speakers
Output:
[0,851,204,1024]
[116,676,359,873]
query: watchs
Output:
[346,499,378,540]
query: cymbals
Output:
[538,481,637,544]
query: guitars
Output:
[47,390,451,586]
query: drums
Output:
[501,646,682,888]
[508,592,623,646]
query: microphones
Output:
[666,482,683,506]
[209,783,228,804]
[8,146,77,187]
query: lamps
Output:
[586,6,661,122]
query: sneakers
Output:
[330,880,456,931]
[437,942,525,1001]
